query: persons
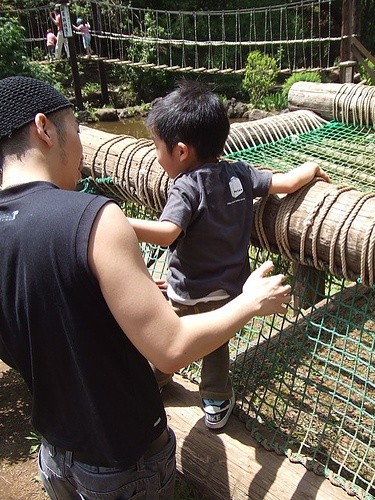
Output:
[50,8,70,60]
[72,19,94,59]
[0,77,292,500]
[125,87,333,429]
[47,30,57,62]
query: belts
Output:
[56,429,169,468]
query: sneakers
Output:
[154,366,173,388]
[202,387,235,428]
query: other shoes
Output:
[90,50,94,54]
[87,53,91,58]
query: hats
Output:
[0,76,75,136]
